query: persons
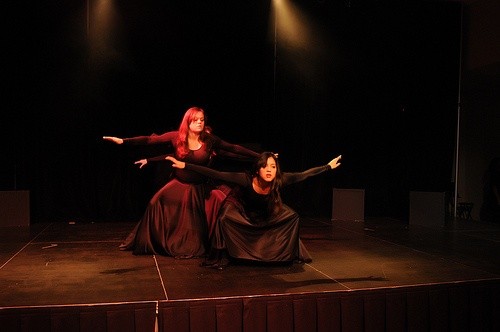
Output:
[166,152,342,266]
[102,107,262,259]
[133,126,261,169]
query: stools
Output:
[458,202,474,225]
